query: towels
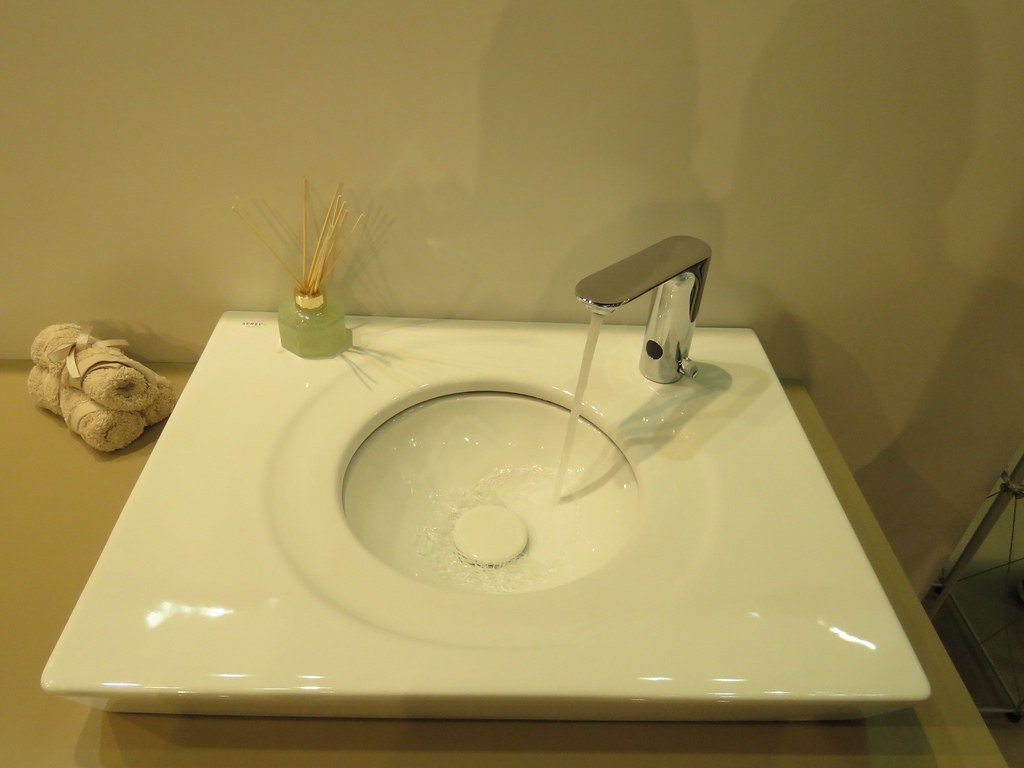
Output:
[27,322,176,452]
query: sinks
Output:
[39,306,934,721]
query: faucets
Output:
[575,235,711,384]
